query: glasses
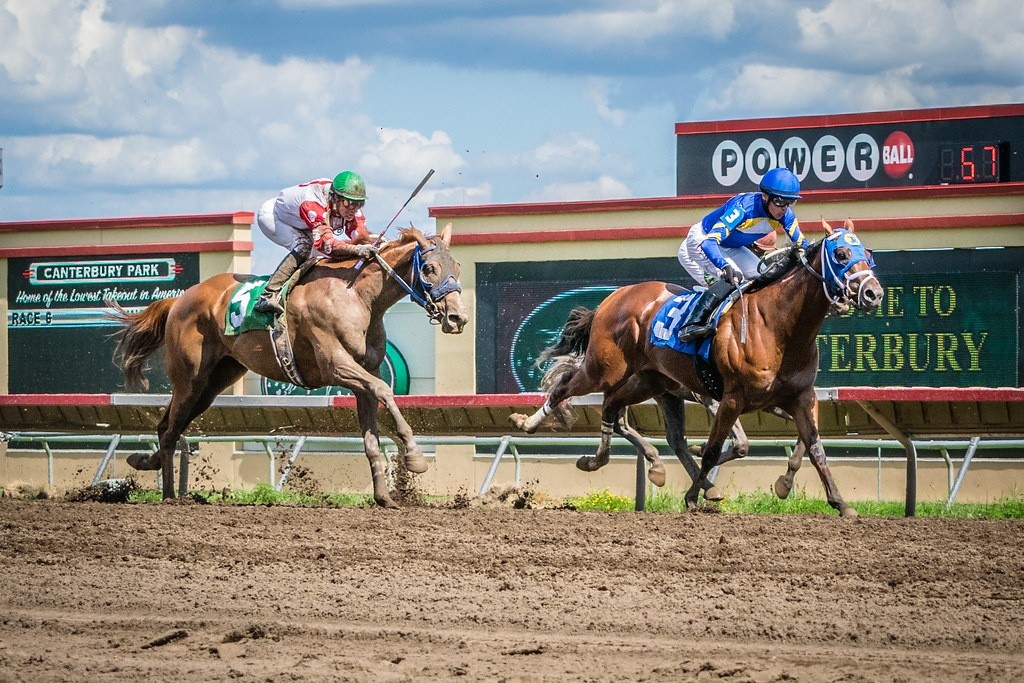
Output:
[341,199,365,210]
[771,196,797,207]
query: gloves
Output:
[358,245,381,260]
[722,265,743,286]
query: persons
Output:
[253,171,388,312]
[678,167,810,342]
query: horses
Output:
[102,221,468,509]
[507,214,883,518]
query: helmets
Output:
[758,167,802,199]
[332,171,369,201]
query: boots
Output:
[678,290,722,342]
[254,249,306,313]
